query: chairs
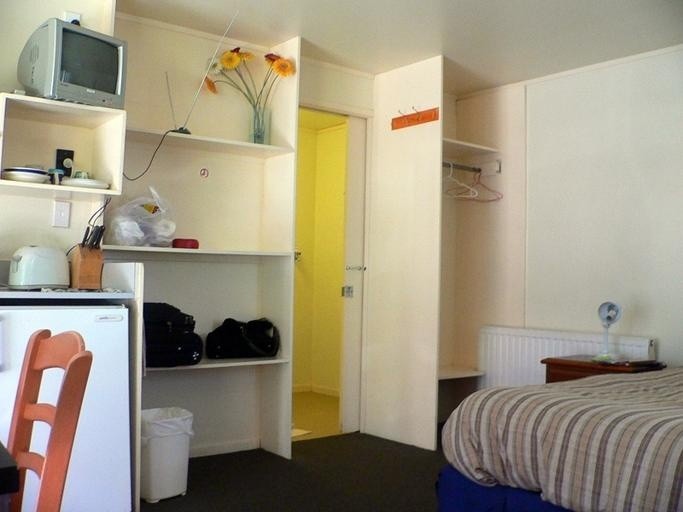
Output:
[0,328,93,512]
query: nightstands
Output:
[539,354,667,384]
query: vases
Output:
[246,107,270,145]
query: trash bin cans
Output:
[140,407,194,503]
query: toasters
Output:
[6,245,71,292]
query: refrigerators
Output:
[0,305,135,512]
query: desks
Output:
[0,441,19,494]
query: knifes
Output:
[80,225,106,250]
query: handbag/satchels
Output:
[206,317,280,360]
[144,302,202,367]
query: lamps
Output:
[597,300,628,364]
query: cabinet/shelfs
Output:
[358,54,528,451]
[0,93,134,302]
[101,11,301,461]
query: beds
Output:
[435,368,683,512]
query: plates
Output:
[59,176,113,190]
[1,165,50,185]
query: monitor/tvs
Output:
[17,17,128,109]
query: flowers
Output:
[202,46,296,144]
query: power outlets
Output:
[63,12,80,26]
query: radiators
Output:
[474,323,658,393]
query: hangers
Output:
[442,161,503,203]
